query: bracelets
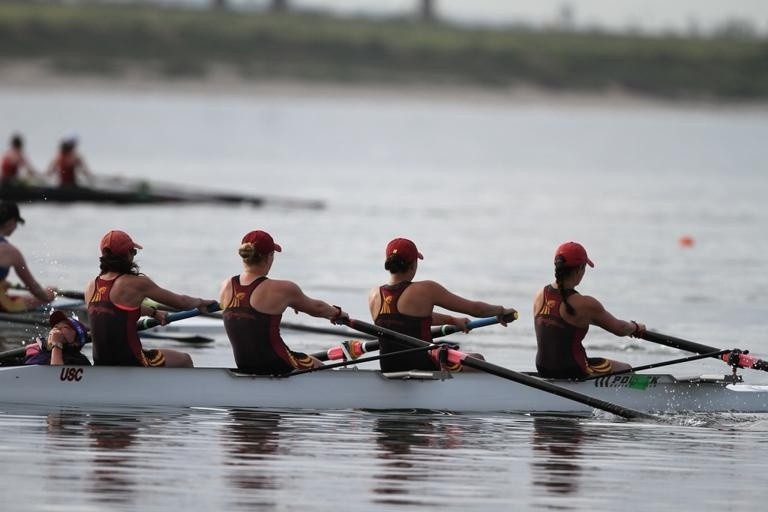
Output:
[50,342,65,350]
[150,306,157,317]
[631,319,639,333]
[333,304,342,318]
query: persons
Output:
[22,310,92,365]
[534,242,646,378]
[0,136,38,192]
[370,238,518,373]
[0,201,57,314]
[45,139,95,193]
[220,231,349,373]
[85,231,218,367]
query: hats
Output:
[100,230,143,254]
[241,230,281,257]
[556,242,594,268]
[0,201,25,223]
[50,310,88,349]
[386,238,423,263]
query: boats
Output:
[17,185,260,204]
[0,362,768,414]
[1,305,90,352]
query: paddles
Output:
[1,312,216,342]
